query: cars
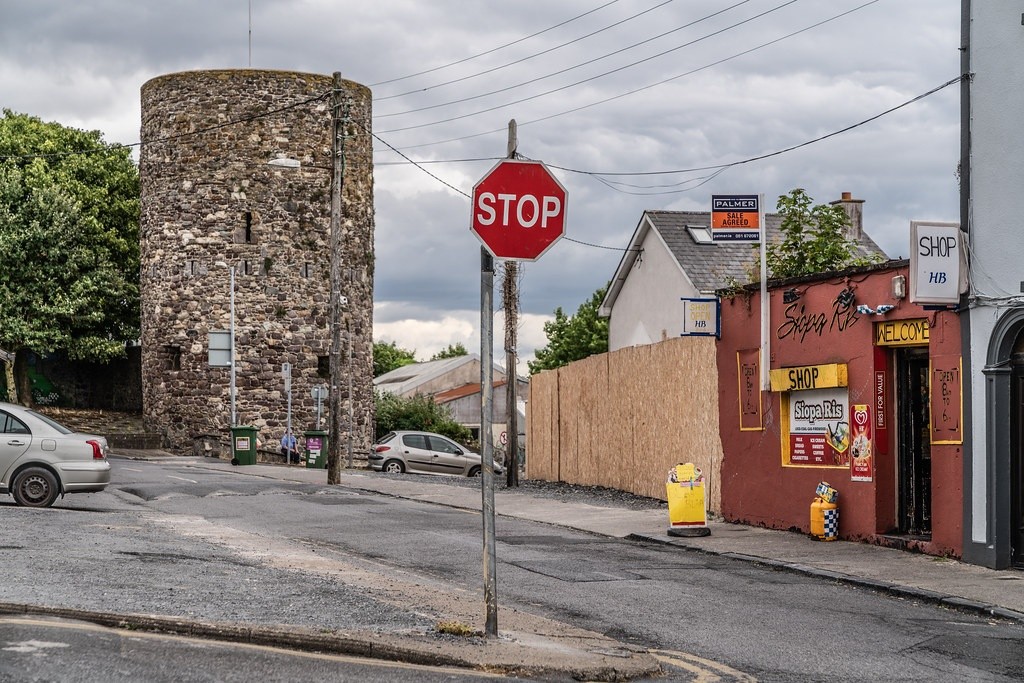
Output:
[0,402,111,508]
[368,431,503,478]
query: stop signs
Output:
[470,159,569,262]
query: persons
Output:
[281,428,304,467]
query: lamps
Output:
[782,288,799,304]
[891,276,905,298]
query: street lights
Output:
[208,261,236,460]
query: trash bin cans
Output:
[230,426,261,467]
[304,430,329,470]
